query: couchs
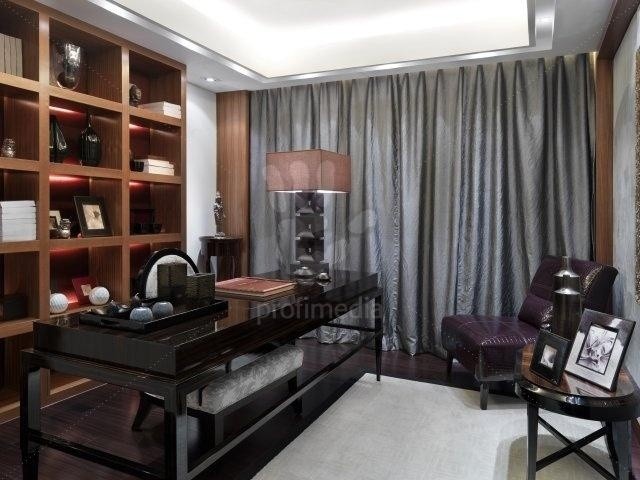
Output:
[441,256,617,410]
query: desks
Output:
[520,344,640,480]
[20,269,385,478]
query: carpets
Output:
[253,370,616,479]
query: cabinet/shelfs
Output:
[0,0,188,426]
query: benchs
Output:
[128,344,305,445]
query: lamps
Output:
[266,150,353,282]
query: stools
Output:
[198,235,241,278]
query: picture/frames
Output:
[564,307,634,393]
[75,195,113,237]
[529,329,570,387]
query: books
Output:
[137,100,182,120]
[0,201,37,242]
[134,155,175,176]
[215,276,297,297]
[0,32,23,77]
